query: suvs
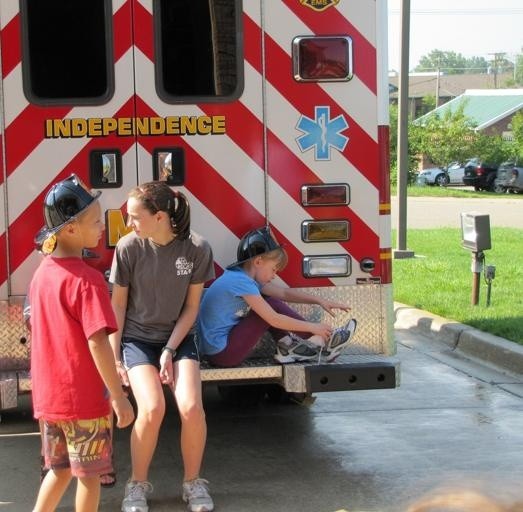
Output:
[494,154,522,194]
[462,156,505,193]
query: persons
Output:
[106,179,218,512]
[22,225,117,489]
[195,225,356,369]
[24,173,138,512]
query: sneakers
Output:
[272,333,321,363]
[181,477,213,512]
[120,477,151,512]
[319,317,357,362]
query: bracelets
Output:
[161,346,177,358]
[108,392,130,401]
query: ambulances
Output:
[0,1,408,418]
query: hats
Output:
[31,172,102,244]
[225,225,283,271]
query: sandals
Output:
[98,465,117,488]
[38,454,49,483]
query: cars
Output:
[413,157,470,187]
